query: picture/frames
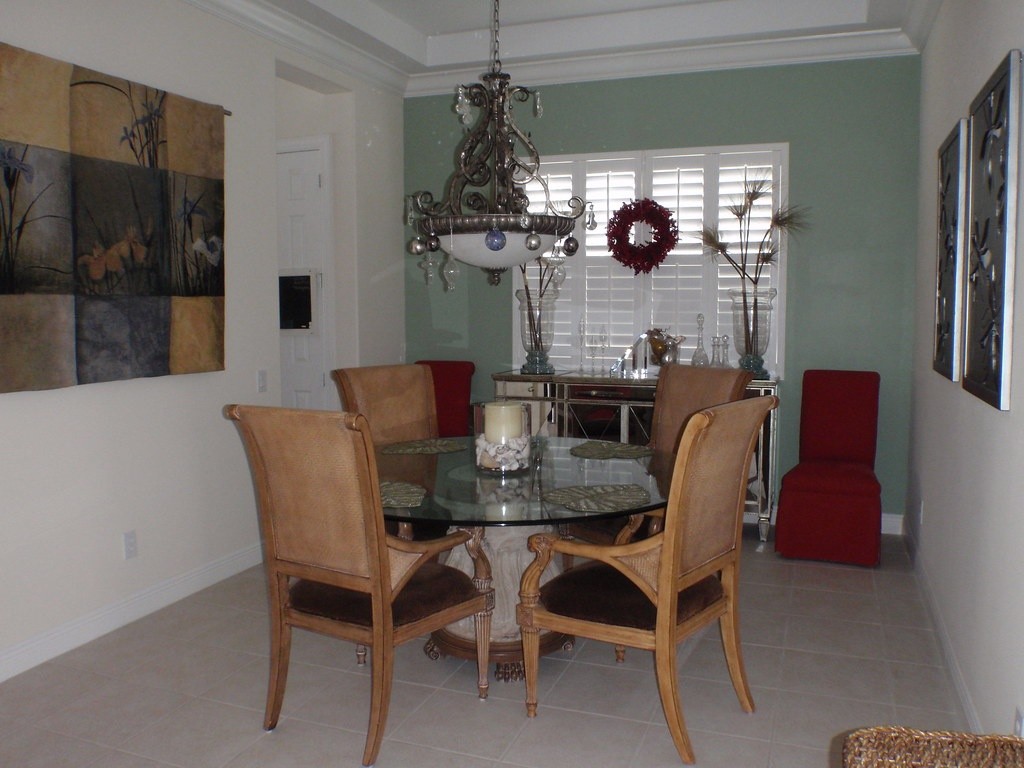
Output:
[933,118,966,382]
[963,49,1021,410]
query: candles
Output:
[484,401,522,445]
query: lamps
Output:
[406,0,597,292]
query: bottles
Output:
[721,335,734,368]
[691,313,709,368]
[710,337,721,368]
[662,339,678,364]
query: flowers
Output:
[605,198,680,276]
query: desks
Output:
[491,368,780,543]
[373,436,677,684]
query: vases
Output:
[728,287,777,380]
[515,289,560,375]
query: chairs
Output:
[415,360,475,438]
[775,370,882,567]
[330,364,450,538]
[516,395,779,764]
[223,404,496,768]
[563,365,754,569]
